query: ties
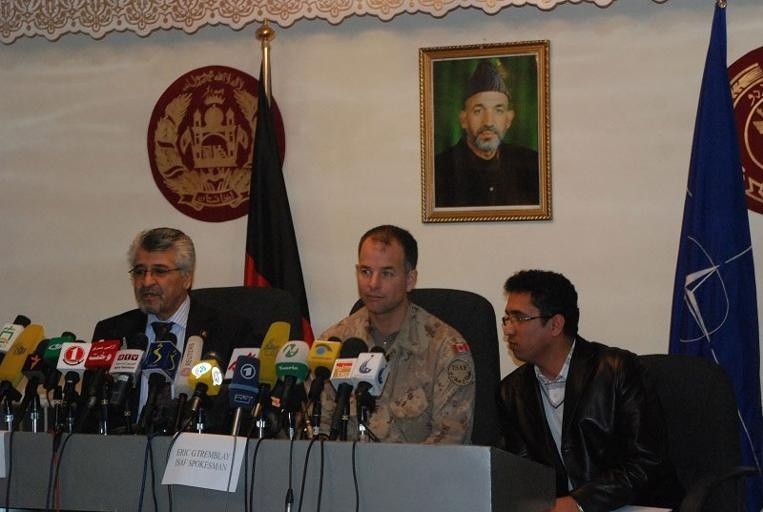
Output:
[149,321,173,416]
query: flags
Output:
[667,0,763,511]
[245,56,316,348]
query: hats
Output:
[462,59,510,101]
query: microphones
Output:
[256,321,291,439]
[14,338,48,433]
[56,340,92,433]
[0,314,31,365]
[0,323,44,431]
[349,346,390,443]
[188,352,224,433]
[41,332,76,432]
[172,336,204,433]
[229,355,259,436]
[329,338,368,441]
[276,340,310,440]
[85,339,122,434]
[307,337,342,439]
[136,333,181,436]
[109,346,145,434]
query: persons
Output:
[296,225,476,445]
[80,227,253,433]
[435,60,538,208]
[494,270,678,512]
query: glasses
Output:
[128,266,183,277]
[502,314,552,324]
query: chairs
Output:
[638,353,762,512]
[341,289,502,426]
[184,288,300,347]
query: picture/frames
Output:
[418,40,552,224]
